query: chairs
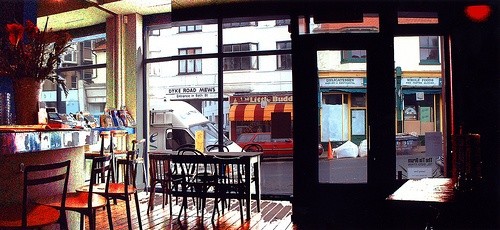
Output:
[147,143,264,223]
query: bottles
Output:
[457,160,487,193]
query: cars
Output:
[235,131,325,161]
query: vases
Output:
[13,77,40,125]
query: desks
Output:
[385,178,460,230]
[149,151,262,220]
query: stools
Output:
[0,131,146,230]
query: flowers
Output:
[0,17,73,99]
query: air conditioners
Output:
[63,51,77,64]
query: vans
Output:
[145,100,256,190]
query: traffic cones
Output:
[327,138,335,160]
